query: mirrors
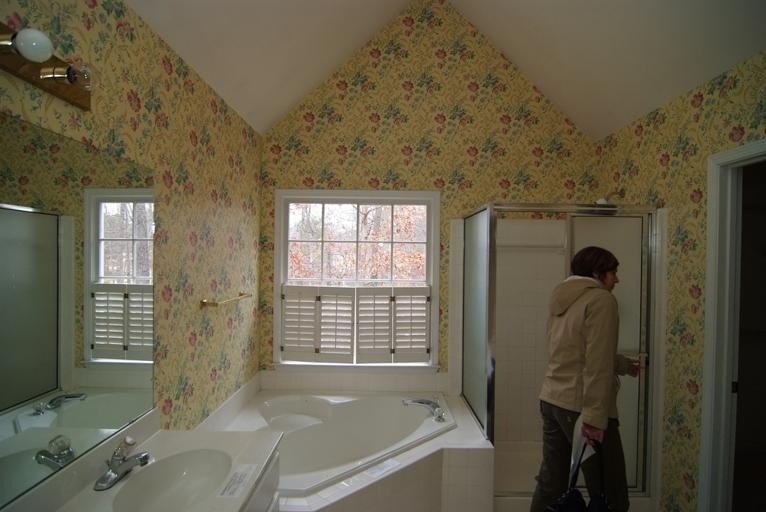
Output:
[0,110,160,512]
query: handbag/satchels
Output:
[542,490,612,511]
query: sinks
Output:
[111,448,232,512]
[0,428,97,507]
[223,389,456,496]
[14,385,154,429]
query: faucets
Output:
[92,436,151,491]
[404,397,443,417]
[34,436,78,467]
[46,390,87,408]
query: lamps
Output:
[1,22,94,114]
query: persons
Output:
[529,246,640,512]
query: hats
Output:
[571,246,619,277]
